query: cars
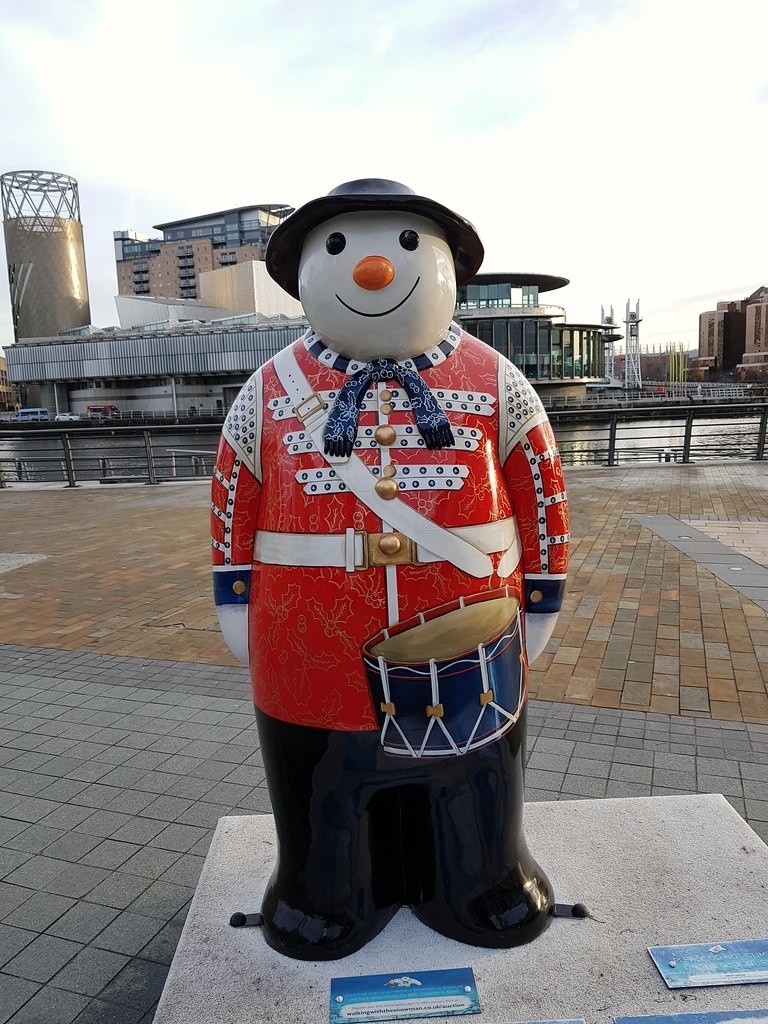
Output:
[55,413,82,421]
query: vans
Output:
[10,408,50,422]
[88,405,120,418]
